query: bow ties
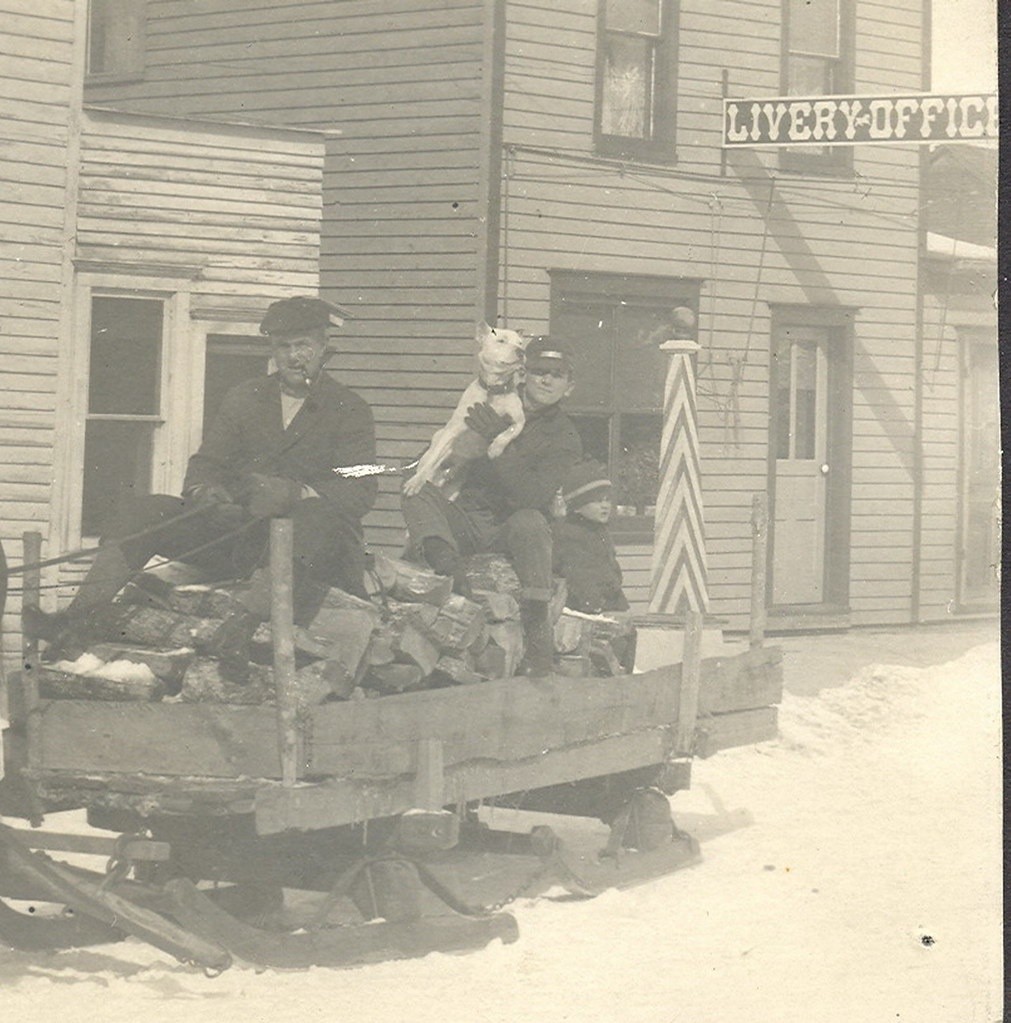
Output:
[282,381,309,397]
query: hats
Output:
[259,298,330,334]
[525,335,568,373]
[561,461,613,508]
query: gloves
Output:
[248,472,302,520]
[192,484,244,526]
[465,402,508,445]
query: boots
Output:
[208,608,260,687]
[22,541,139,662]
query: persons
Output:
[23,297,379,659]
[402,334,637,678]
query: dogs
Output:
[399,316,529,507]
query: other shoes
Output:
[424,539,471,599]
[525,620,554,678]
